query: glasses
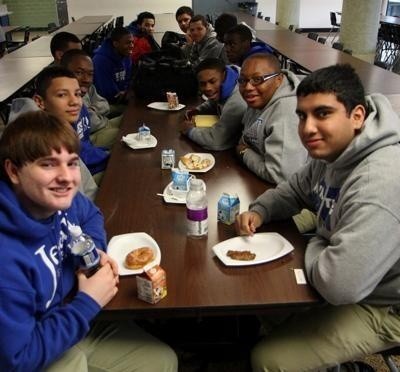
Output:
[236,72,280,87]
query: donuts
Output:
[125,248,154,268]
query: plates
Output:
[145,100,187,111]
[178,151,217,172]
[124,132,157,150]
[164,178,206,207]
[211,231,295,266]
[106,231,162,277]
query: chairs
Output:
[206,8,400,74]
[0,16,128,130]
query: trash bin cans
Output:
[238,2,258,15]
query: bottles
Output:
[68,224,102,274]
[184,180,208,240]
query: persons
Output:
[234,64,399,372]
[233,52,312,185]
[1,110,178,372]
[50,6,277,174]
[1,64,103,205]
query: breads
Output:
[179,155,209,170]
